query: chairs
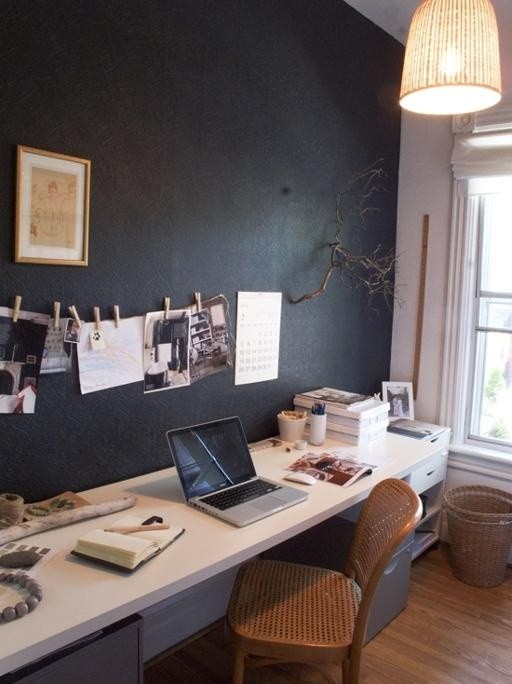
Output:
[222,475,424,683]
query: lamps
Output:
[396,0,506,116]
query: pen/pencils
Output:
[312,401,325,415]
[104,523,170,531]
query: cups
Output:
[309,411,327,445]
[293,439,307,449]
[0,493,26,529]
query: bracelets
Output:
[0,570,43,623]
[28,503,52,516]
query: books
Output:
[69,514,186,573]
[282,386,391,489]
[385,417,445,442]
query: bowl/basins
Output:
[277,414,308,442]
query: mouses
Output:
[284,472,317,485]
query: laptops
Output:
[166,416,310,527]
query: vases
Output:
[276,410,309,443]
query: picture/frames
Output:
[380,379,416,422]
[12,141,94,269]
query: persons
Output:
[65,321,80,342]
[393,394,404,418]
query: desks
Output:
[0,417,453,682]
